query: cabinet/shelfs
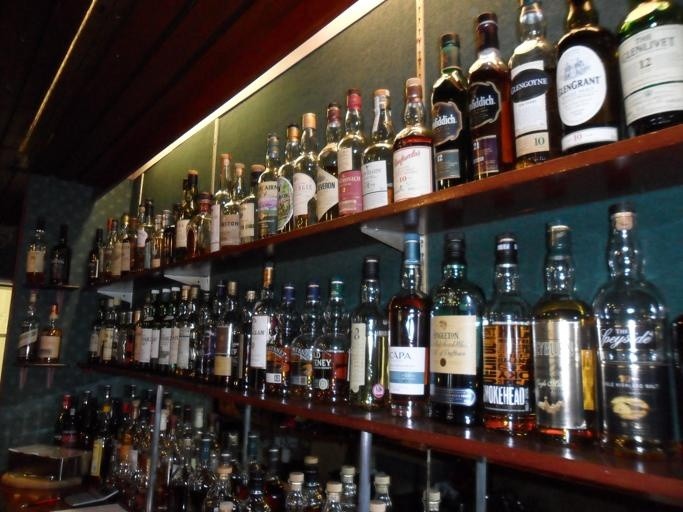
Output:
[10,279,79,369]
[76,119,682,512]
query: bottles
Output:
[15,1,683,510]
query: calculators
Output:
[63,485,120,508]
